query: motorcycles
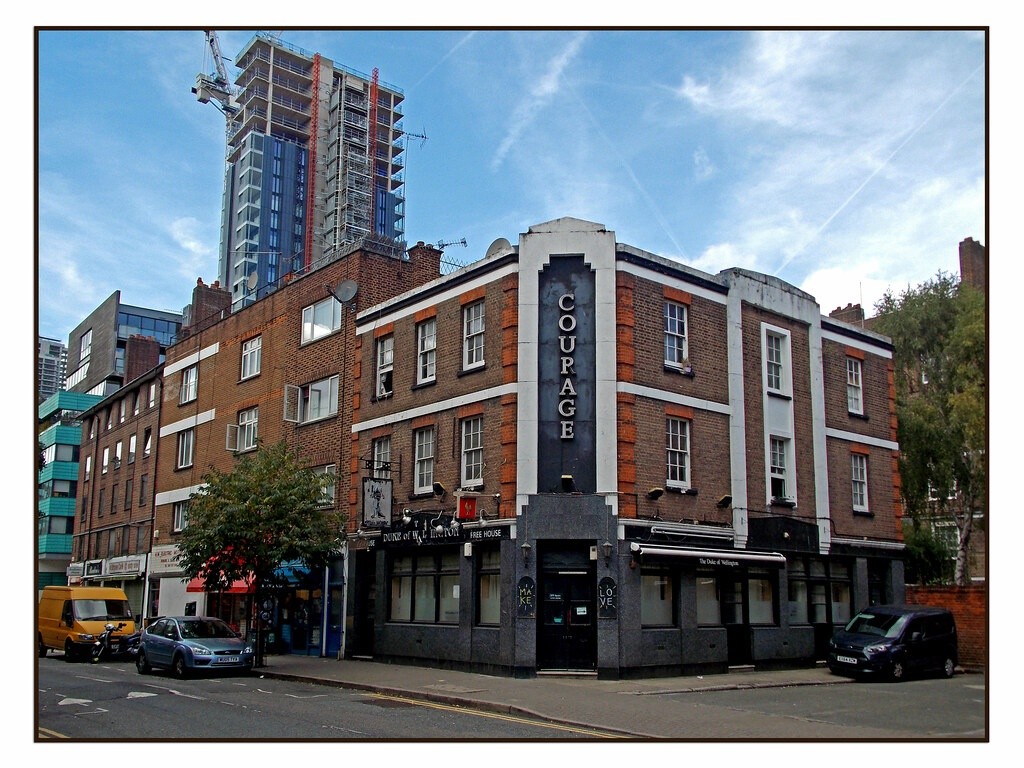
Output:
[89,616,166,664]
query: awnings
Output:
[186,570,257,593]
[629,541,786,571]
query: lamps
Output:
[451,510,460,528]
[519,506,531,565]
[479,509,498,526]
[717,495,732,507]
[432,510,444,533]
[433,482,448,502]
[603,505,613,566]
[563,473,574,488]
[402,507,420,523]
[647,487,664,500]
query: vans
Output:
[826,605,959,682]
[39,586,141,663]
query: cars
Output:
[136,616,253,679]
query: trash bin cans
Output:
[144,615,166,631]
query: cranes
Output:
[192,30,245,291]
